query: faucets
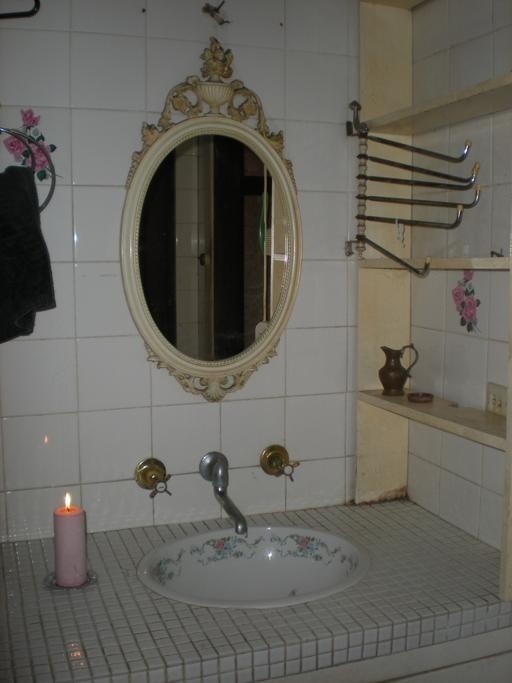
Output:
[198,451,248,535]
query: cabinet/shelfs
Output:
[349,0,511,602]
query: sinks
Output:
[136,525,371,608]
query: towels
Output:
[0,164,59,344]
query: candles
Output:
[54,491,90,588]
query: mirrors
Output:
[121,34,304,404]
